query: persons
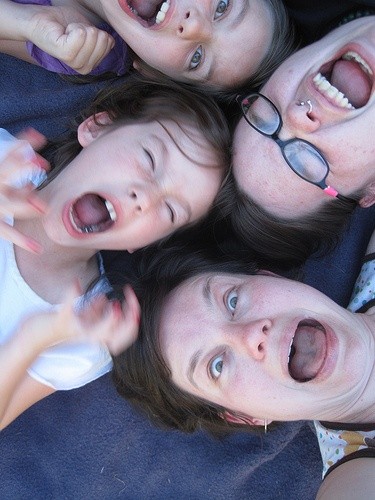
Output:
[111,231,375,500]
[214,17,375,258]
[0,60,233,430]
[0,0,292,101]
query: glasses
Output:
[236,90,357,206]
